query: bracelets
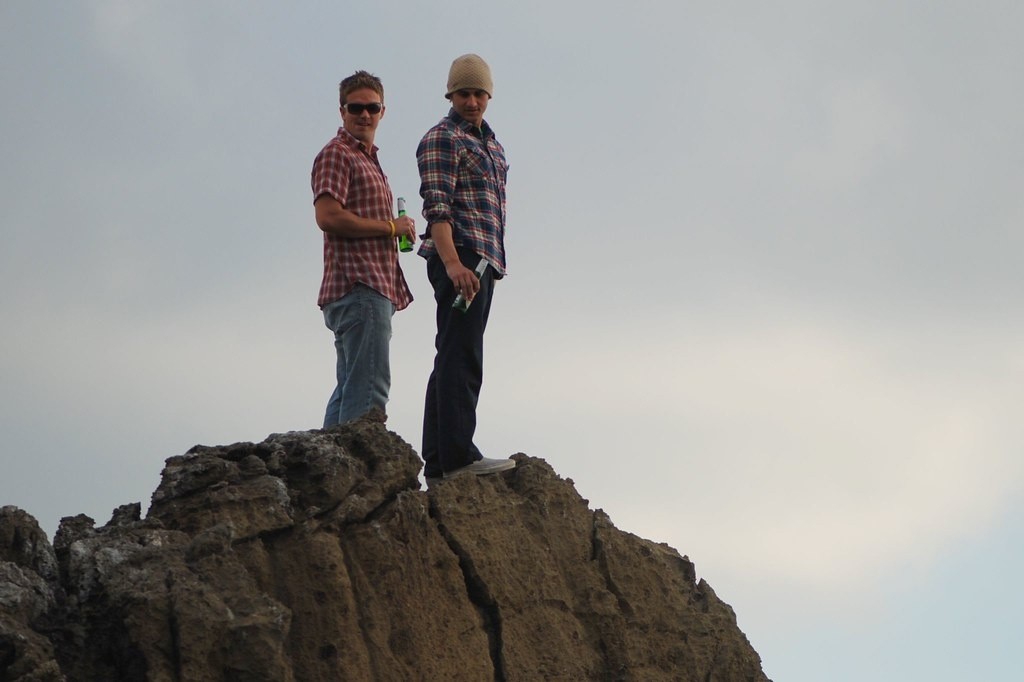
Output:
[388,221,395,238]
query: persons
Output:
[311,70,416,428]
[417,53,516,487]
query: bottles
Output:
[452,259,490,311]
[399,197,413,253]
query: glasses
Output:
[344,102,382,115]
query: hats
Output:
[445,53,493,99]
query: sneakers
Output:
[442,455,515,478]
[425,475,445,486]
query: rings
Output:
[412,223,413,225]
